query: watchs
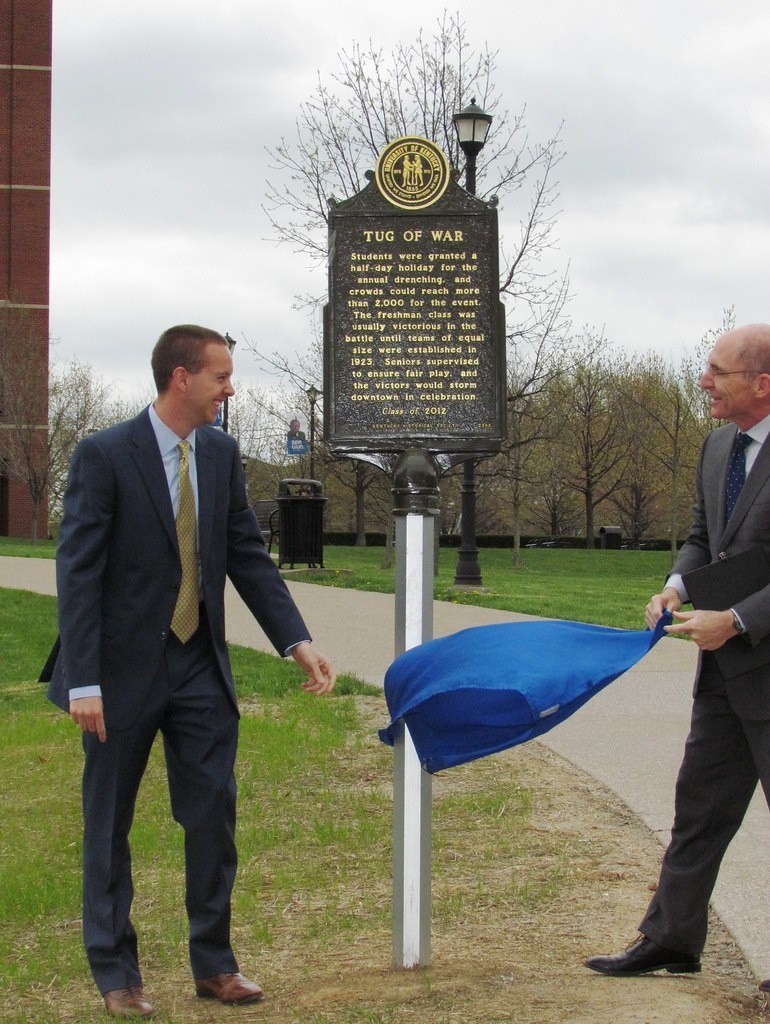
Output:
[731,611,744,635]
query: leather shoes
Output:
[194,972,263,1006]
[102,985,154,1020]
[580,932,702,978]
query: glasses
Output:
[704,359,762,379]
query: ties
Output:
[724,433,754,532]
[169,439,200,645]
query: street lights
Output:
[454,96,493,585]
[221,331,236,434]
[305,383,321,480]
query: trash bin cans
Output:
[277,478,328,570]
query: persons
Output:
[582,321,770,994]
[34,324,341,1021]
[287,419,306,441]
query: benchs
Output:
[251,500,280,556]
